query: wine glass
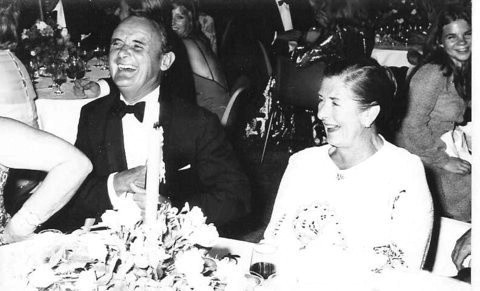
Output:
[249,247,278,291]
[66,59,86,80]
[52,67,67,95]
[93,43,106,69]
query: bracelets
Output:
[19,209,42,227]
[23,204,44,225]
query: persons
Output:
[0,8,39,216]
[0,0,321,98]
[59,13,261,230]
[258,57,434,271]
[0,116,93,246]
[170,0,231,123]
[393,6,471,223]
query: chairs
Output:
[220,87,248,128]
[258,57,326,167]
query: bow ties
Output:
[113,99,145,122]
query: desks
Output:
[371,40,416,81]
[0,237,259,291]
[35,65,110,145]
